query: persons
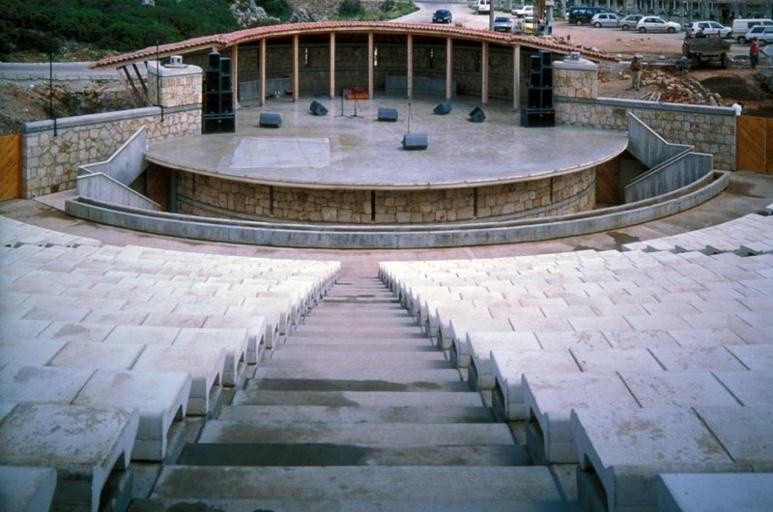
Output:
[731,99,744,118]
[629,56,641,92]
[749,36,760,69]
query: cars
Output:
[477,0,546,34]
[567,8,773,68]
[432,9,451,25]
